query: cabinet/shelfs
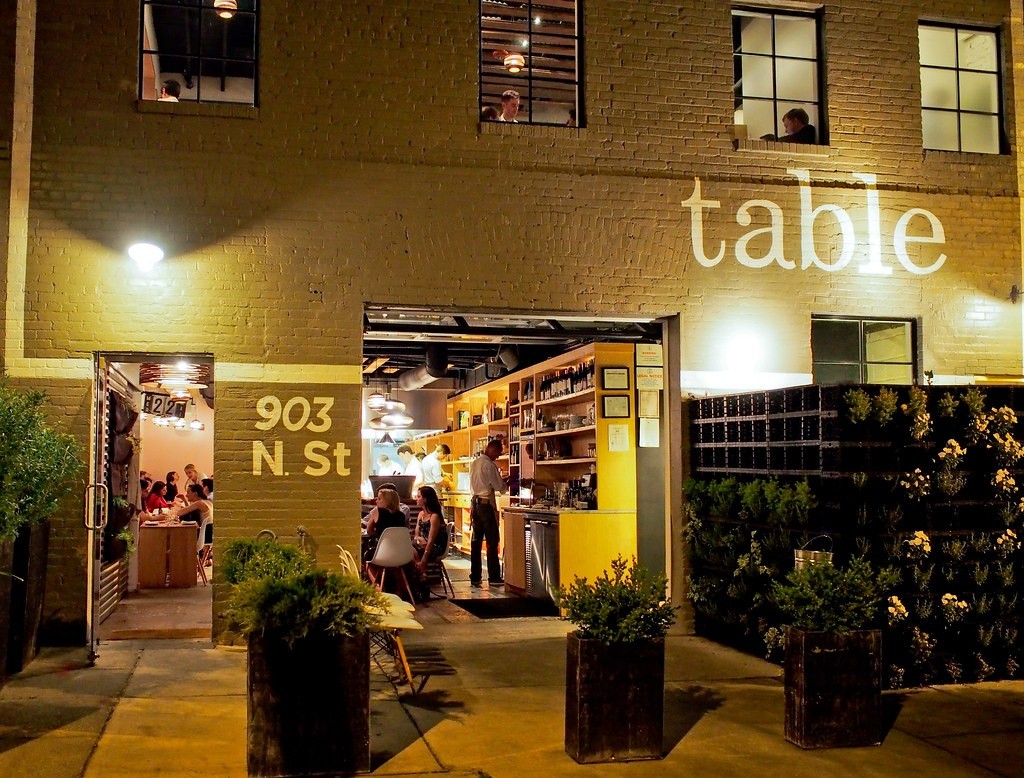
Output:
[407,343,635,618]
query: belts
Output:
[473,498,489,504]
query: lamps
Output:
[504,18,525,73]
[364,346,516,446]
[213,0,238,18]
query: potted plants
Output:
[765,556,901,750]
[564,553,682,761]
[227,534,380,778]
[1,371,90,681]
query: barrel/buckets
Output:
[795,535,836,573]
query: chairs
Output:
[334,543,424,697]
[196,522,214,584]
[366,527,415,605]
[426,522,456,598]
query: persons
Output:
[377,455,403,476]
[146,481,184,513]
[366,489,405,597]
[499,90,520,122]
[567,109,576,126]
[415,452,427,462]
[184,464,208,492]
[760,134,776,141]
[136,479,164,524]
[469,440,511,586]
[201,479,213,500]
[140,471,148,479]
[361,483,410,530]
[422,444,450,520]
[158,80,181,102]
[482,107,499,120]
[177,484,213,552]
[402,486,448,601]
[397,445,423,500]
[163,471,180,502]
[778,108,816,143]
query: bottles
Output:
[567,479,588,508]
[158,503,163,515]
[508,360,596,464]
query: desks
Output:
[139,519,199,588]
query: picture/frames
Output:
[602,367,630,390]
[603,395,630,419]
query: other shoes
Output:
[419,568,428,585]
[489,578,504,585]
[471,581,480,587]
